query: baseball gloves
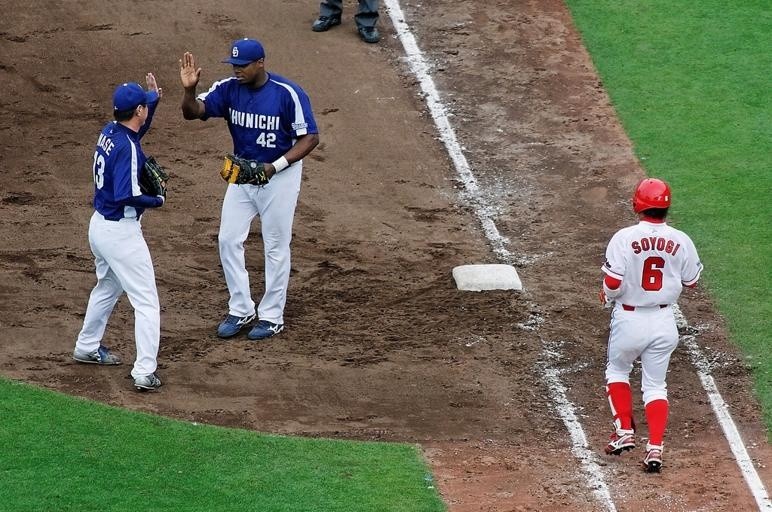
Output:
[139,156,168,199]
[221,152,269,186]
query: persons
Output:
[311,0,386,44]
[593,176,704,475]
[71,72,171,393]
[175,36,320,340]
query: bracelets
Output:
[271,155,290,174]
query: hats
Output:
[114,82,157,110]
[221,38,265,65]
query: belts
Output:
[622,303,668,311]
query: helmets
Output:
[633,178,671,213]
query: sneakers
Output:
[606,430,664,472]
[134,374,162,389]
[72,345,122,365]
[313,14,342,32]
[360,27,379,43]
[217,314,285,339]
